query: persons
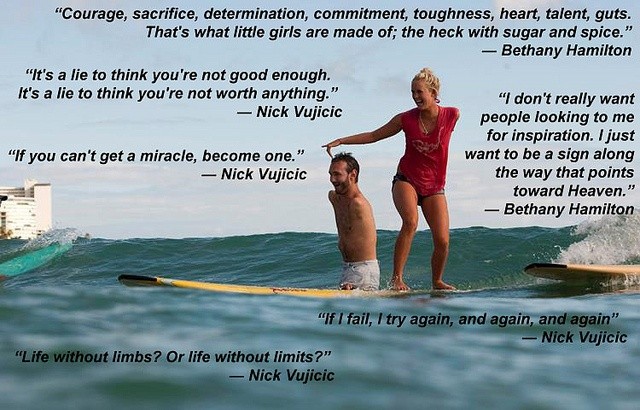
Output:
[322,67,460,291]
[328,152,380,291]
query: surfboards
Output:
[118,273,495,300]
[524,263,639,283]
